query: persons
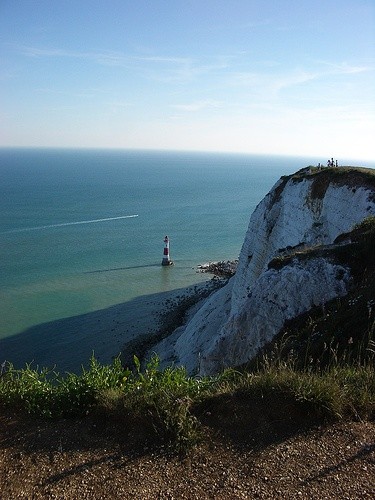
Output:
[336,160,338,167]
[327,160,331,167]
[331,158,334,167]
